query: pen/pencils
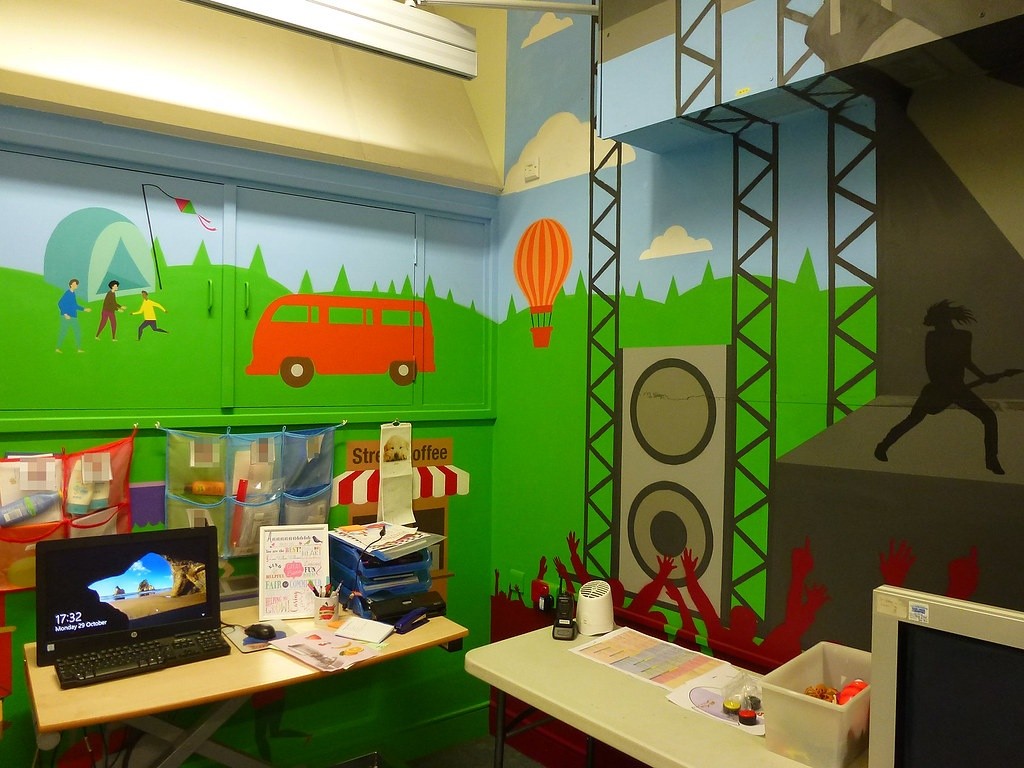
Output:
[307,575,342,597]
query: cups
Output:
[312,591,340,625]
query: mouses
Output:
[244,623,277,639]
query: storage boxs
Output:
[758,640,872,768]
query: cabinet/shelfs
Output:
[331,527,434,623]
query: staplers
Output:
[394,607,429,634]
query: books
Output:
[328,522,446,559]
[335,616,395,643]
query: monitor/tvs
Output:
[35,526,222,667]
[870,584,1024,767]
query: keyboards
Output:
[53,629,231,688]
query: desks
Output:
[23,605,469,768]
[465,618,869,768]
